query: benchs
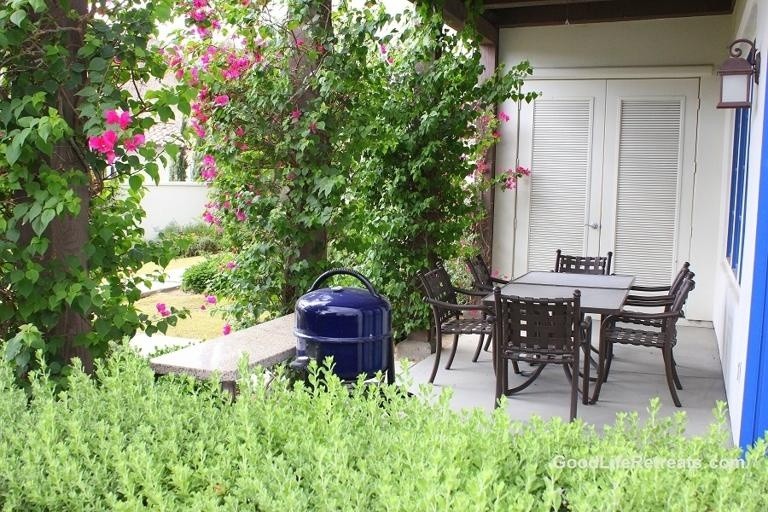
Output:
[149,313,298,400]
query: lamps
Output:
[715,38,761,108]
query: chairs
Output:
[416,249,694,425]
